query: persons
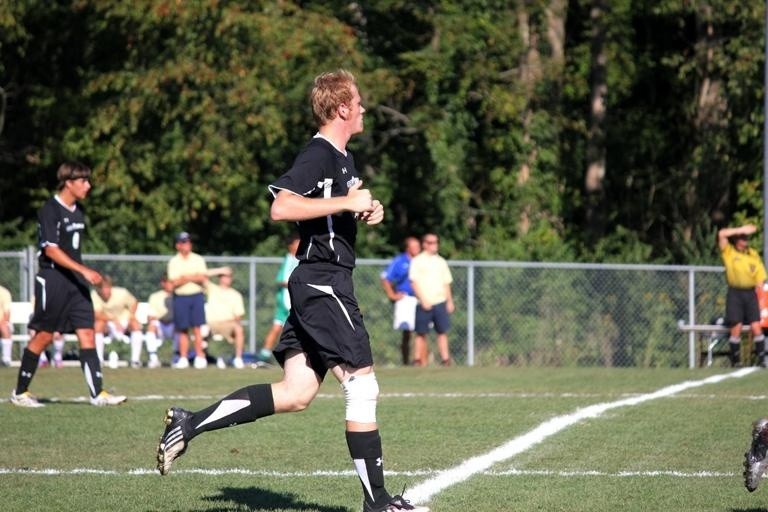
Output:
[754,281,768,348]
[408,233,455,368]
[29,273,245,368]
[258,231,301,362]
[161,232,209,368]
[379,237,429,366]
[156,68,430,512]
[718,222,768,367]
[1,285,12,367]
[10,159,128,409]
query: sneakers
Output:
[743,419,768,492]
[157,407,194,475]
[90,391,126,406]
[364,483,429,512]
[175,357,243,368]
[10,390,44,408]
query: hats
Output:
[176,232,190,242]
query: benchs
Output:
[678,320,753,369]
[0,301,239,369]
